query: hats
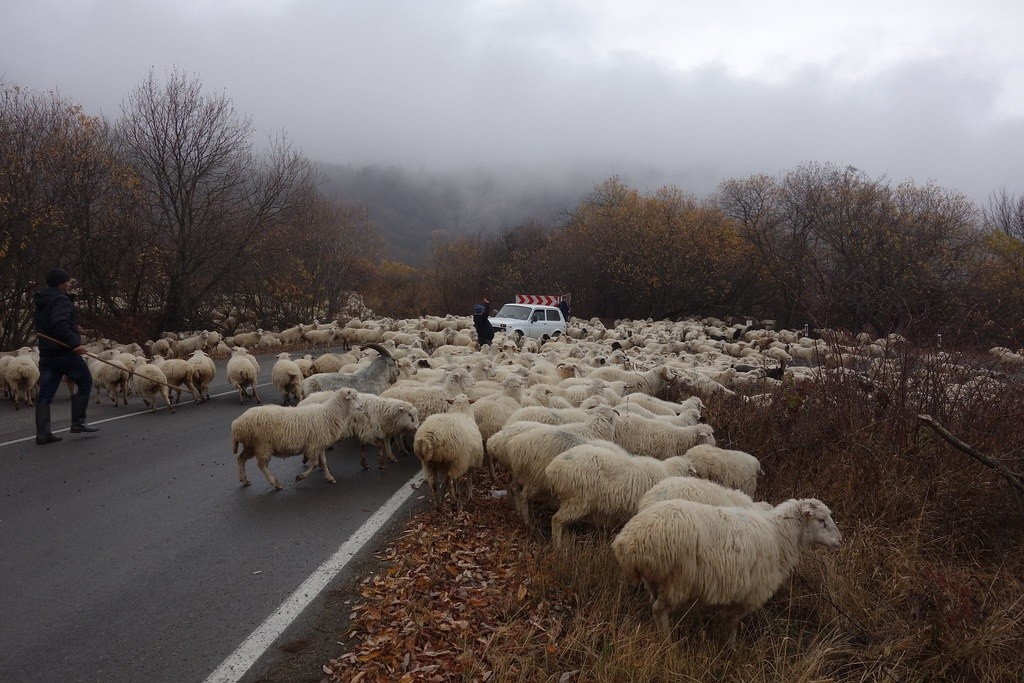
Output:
[46,267,71,287]
[474,304,485,314]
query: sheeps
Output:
[0,287,1024,553]
[231,389,359,490]
[611,489,843,652]
[226,344,260,404]
[414,392,483,513]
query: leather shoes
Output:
[46,432,64,442]
[71,421,100,433]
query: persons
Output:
[34,267,100,445]
[474,298,494,347]
[560,296,570,323]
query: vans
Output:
[486,303,567,338]
